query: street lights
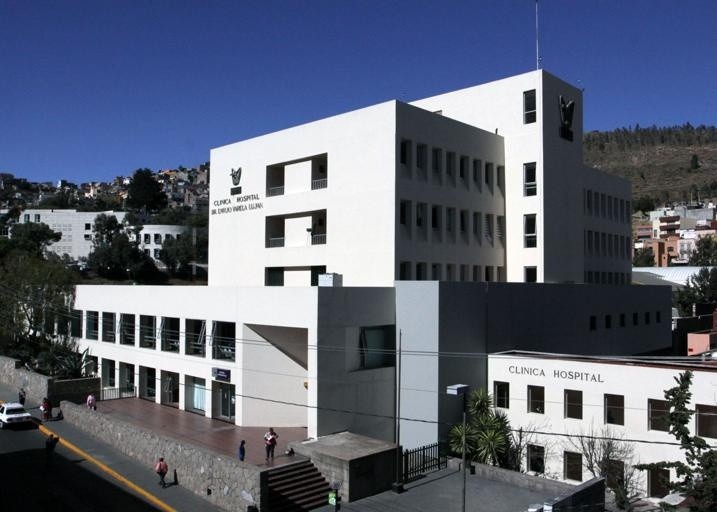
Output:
[445,384,469,511]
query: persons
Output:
[267,432,277,449]
[18,387,25,406]
[86,393,95,411]
[264,428,278,462]
[154,454,168,488]
[45,433,55,457]
[39,397,48,423]
[238,439,245,462]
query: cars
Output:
[0,402,33,430]
